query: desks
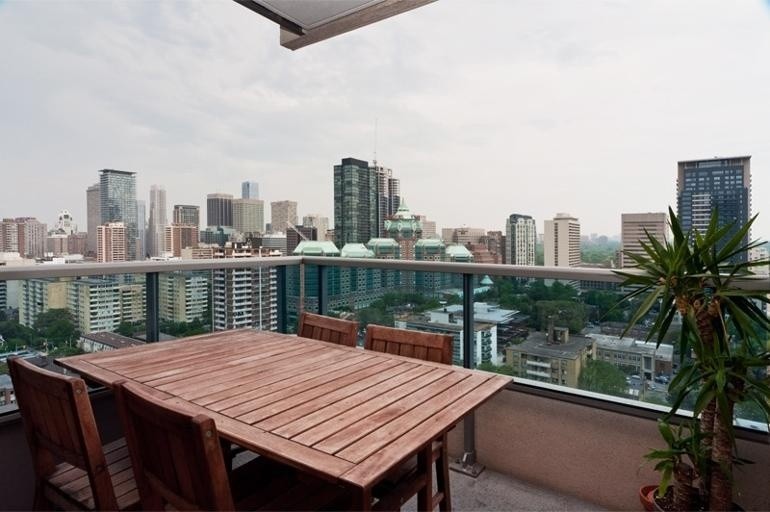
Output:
[51,323,519,512]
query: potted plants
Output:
[610,205,770,512]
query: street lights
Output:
[13,334,71,354]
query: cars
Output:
[625,373,655,391]
[654,373,671,385]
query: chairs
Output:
[6,349,143,511]
[360,320,460,512]
[297,308,365,351]
[111,375,386,511]
